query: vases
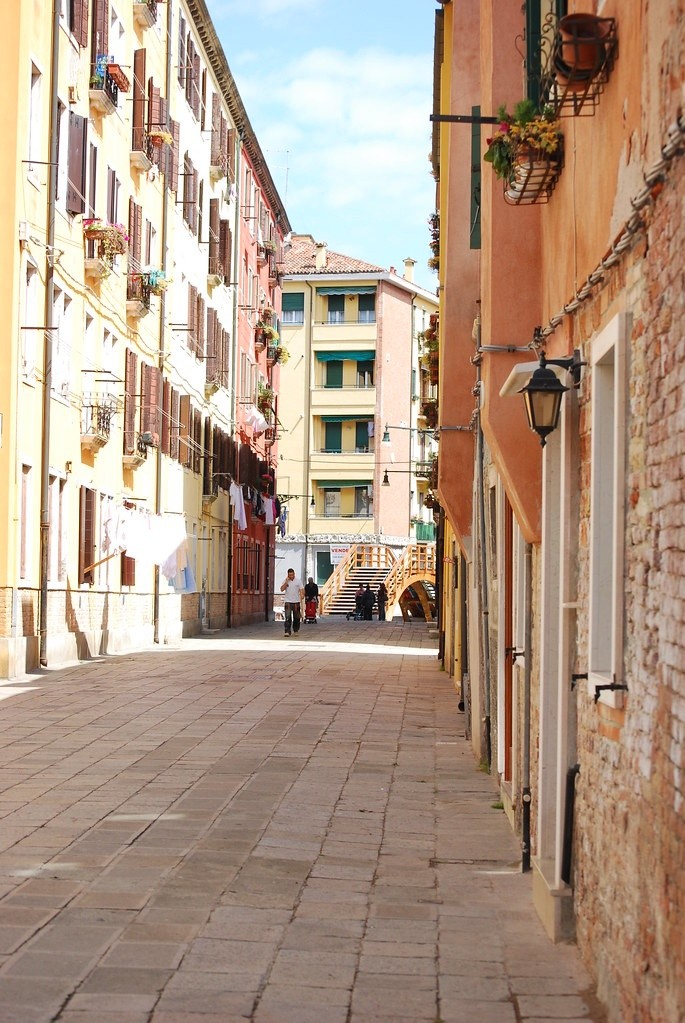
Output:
[553,14,607,91]
[498,143,557,201]
[108,64,129,92]
[87,230,109,240]
[430,220,439,269]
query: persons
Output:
[281,569,305,637]
[355,583,388,621]
[304,578,319,624]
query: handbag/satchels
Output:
[384,594,388,600]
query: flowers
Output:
[484,99,558,189]
[85,220,130,279]
[427,210,440,270]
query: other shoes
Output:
[294,632,299,636]
[284,633,290,637]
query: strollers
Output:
[303,597,318,624]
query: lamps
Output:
[382,426,438,442]
[516,349,581,448]
[382,469,438,486]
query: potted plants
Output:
[418,328,439,385]
[149,132,172,147]
[424,493,434,508]
[268,240,276,254]
[259,388,274,410]
[276,346,290,366]
[265,327,279,341]
[421,402,436,428]
[265,307,275,318]
